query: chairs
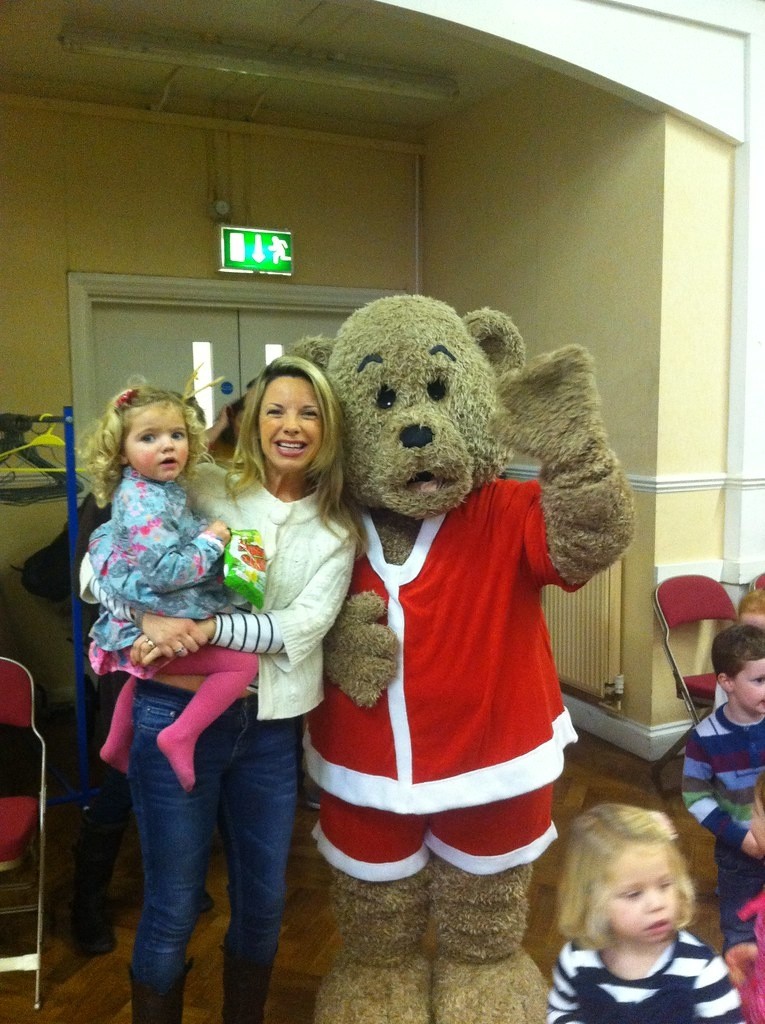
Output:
[0,653,48,1011]
[650,574,739,774]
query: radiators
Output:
[540,555,625,714]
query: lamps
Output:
[59,21,462,108]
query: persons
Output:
[546,802,743,1024]
[79,352,364,1024]
[78,361,258,791]
[21,395,323,959]
[682,587,765,1024]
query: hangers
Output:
[0,413,92,506]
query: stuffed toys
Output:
[294,294,637,1024]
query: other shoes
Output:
[304,783,320,811]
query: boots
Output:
[71,818,130,955]
[219,930,278,1024]
[199,890,214,912]
[128,955,194,1024]
